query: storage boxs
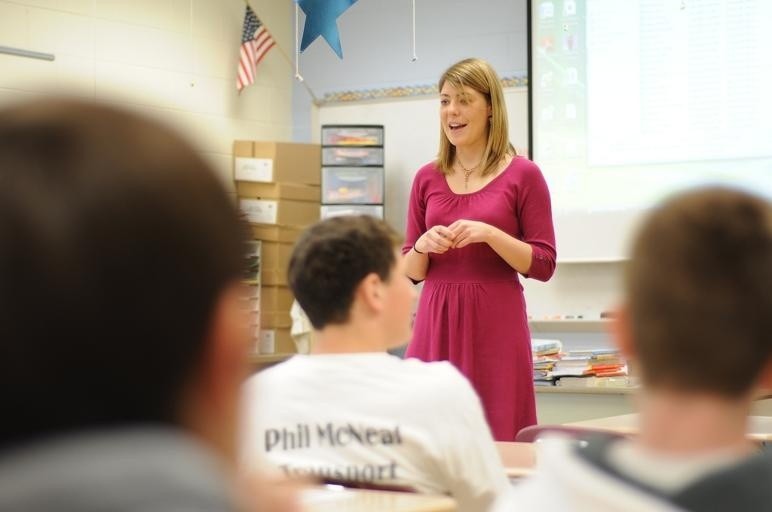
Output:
[232,123,384,355]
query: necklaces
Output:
[452,155,483,192]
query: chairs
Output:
[513,426,626,443]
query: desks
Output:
[494,441,534,481]
[234,354,294,366]
[275,483,457,512]
[532,387,770,426]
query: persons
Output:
[485,175,772,511]
[397,53,562,446]
[228,206,520,512]
[0,82,311,512]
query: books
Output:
[523,337,628,389]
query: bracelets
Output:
[412,238,425,256]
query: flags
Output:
[233,3,276,97]
